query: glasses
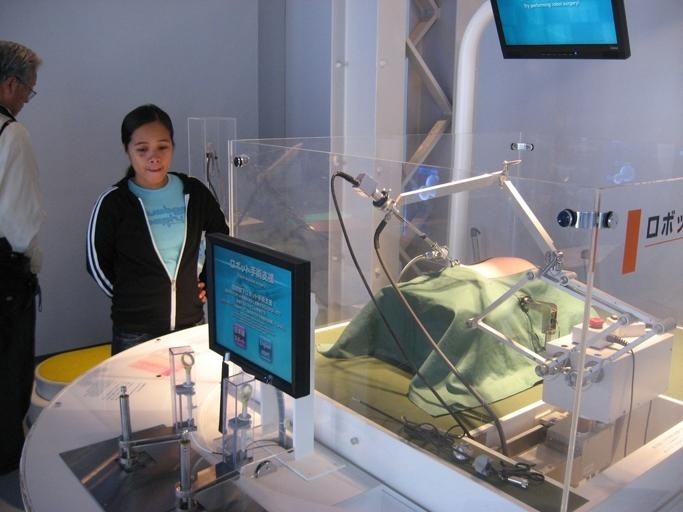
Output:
[15,78,38,100]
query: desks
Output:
[17,320,541,512]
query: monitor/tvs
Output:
[488,0,632,61]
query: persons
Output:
[85,104,230,357]
[0,42,53,477]
